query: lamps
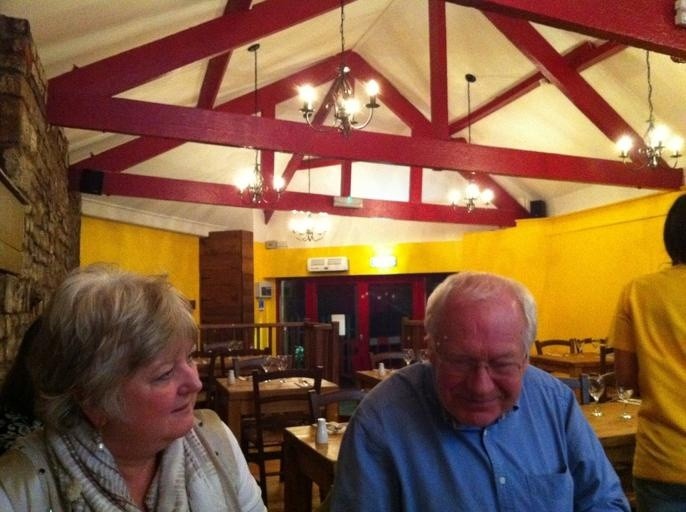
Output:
[290,0,383,140]
[613,50,685,171]
[232,43,286,207]
[448,74,498,214]
[289,154,328,242]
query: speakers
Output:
[531,200,545,217]
[79,168,105,195]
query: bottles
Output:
[570,338,582,354]
[377,361,384,376]
[315,418,329,445]
[227,370,235,385]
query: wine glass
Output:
[588,376,606,417]
[616,384,634,421]
[402,349,414,366]
[257,355,289,384]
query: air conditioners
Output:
[307,257,348,272]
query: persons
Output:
[316,270,633,511]
[0,262,272,512]
[607,193,685,511]
[0,311,46,455]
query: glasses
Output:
[431,342,529,379]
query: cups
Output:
[419,349,431,362]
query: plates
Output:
[313,420,347,435]
